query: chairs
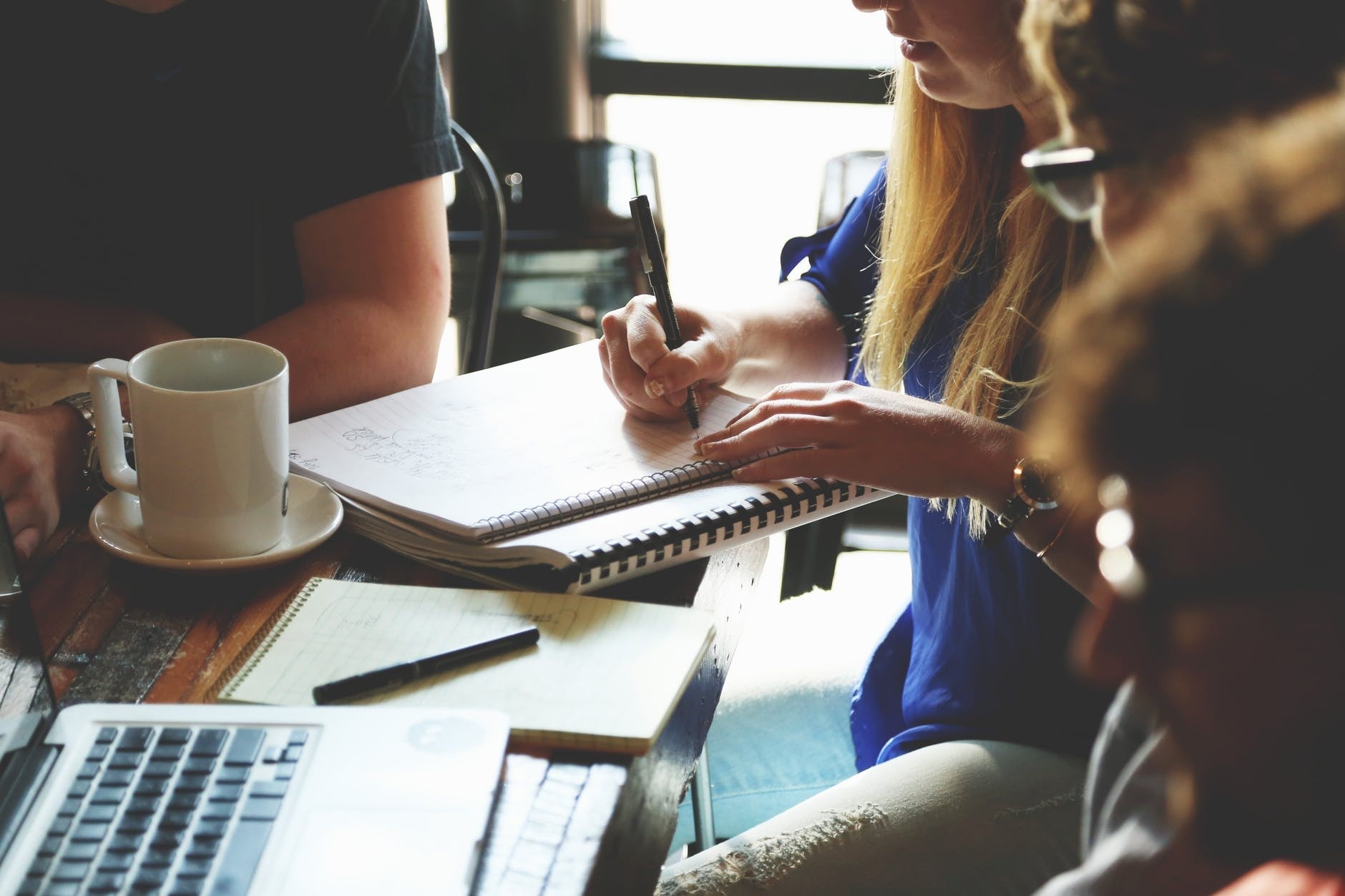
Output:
[450,120,665,375]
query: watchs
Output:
[53,392,137,495]
[998,453,1068,530]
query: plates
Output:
[89,471,344,576]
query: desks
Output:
[15,511,769,895]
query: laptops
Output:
[1,501,509,896]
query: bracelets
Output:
[1036,500,1083,558]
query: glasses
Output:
[1018,126,1148,222]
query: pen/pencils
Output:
[632,195,704,440]
[310,622,542,705]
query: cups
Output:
[85,336,291,560]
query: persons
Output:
[1018,1,1345,857]
[1040,99,1345,896]
[1,0,466,559]
[598,0,1110,896]
